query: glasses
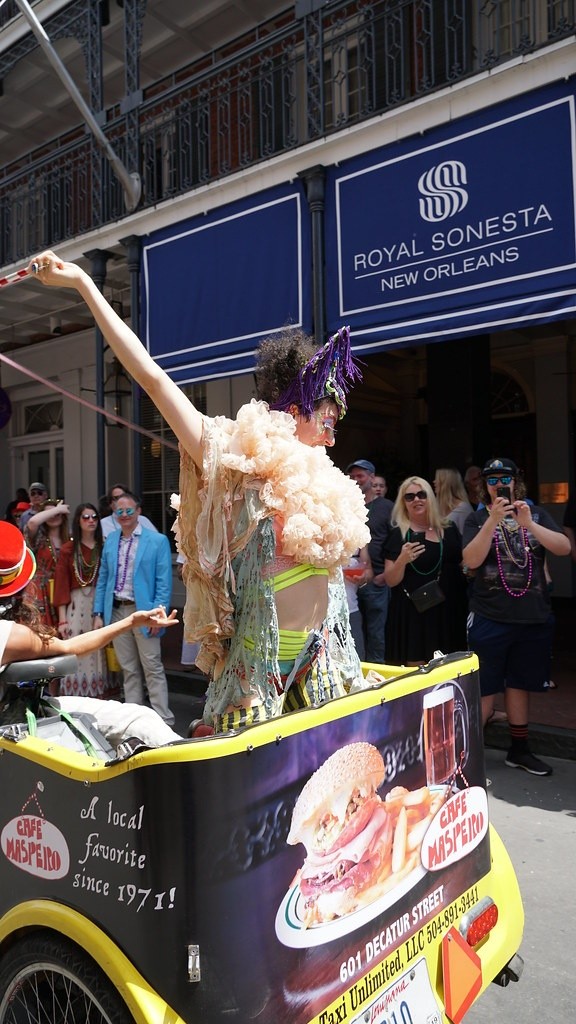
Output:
[404,490,427,501]
[488,476,515,485]
[31,491,44,496]
[82,514,98,521]
[112,493,124,500]
[116,508,136,516]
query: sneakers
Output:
[505,746,554,775]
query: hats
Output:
[30,482,48,490]
[483,458,517,476]
[345,460,375,473]
[0,521,36,597]
[12,502,30,514]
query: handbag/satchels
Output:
[410,580,445,613]
[106,641,121,672]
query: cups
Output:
[423,685,469,787]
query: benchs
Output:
[22,650,485,856]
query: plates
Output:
[274,785,460,949]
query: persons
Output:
[466,497,557,689]
[6,483,71,696]
[28,249,372,734]
[53,504,122,701]
[99,484,161,544]
[92,493,175,729]
[562,496,576,563]
[434,466,483,536]
[462,458,572,774]
[1,519,180,668]
[343,460,395,662]
[381,477,467,666]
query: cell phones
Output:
[497,487,511,507]
[412,531,425,552]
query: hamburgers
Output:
[287,741,395,925]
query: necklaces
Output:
[487,504,532,596]
[47,536,57,562]
[73,539,99,597]
[408,528,443,575]
[115,532,133,591]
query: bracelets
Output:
[92,612,103,618]
[59,621,68,625]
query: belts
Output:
[114,600,133,606]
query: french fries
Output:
[355,785,447,905]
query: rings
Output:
[32,263,45,274]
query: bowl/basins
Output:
[342,561,367,577]
